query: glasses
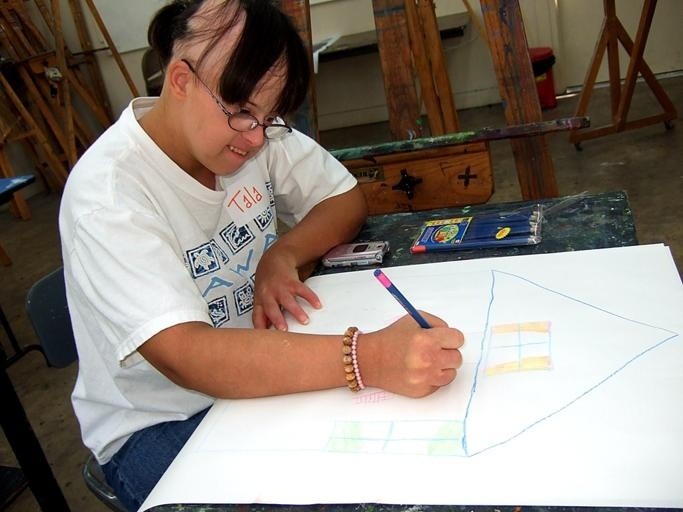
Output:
[182,60,294,141]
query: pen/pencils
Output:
[374,270,431,329]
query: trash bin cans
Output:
[527,47,558,110]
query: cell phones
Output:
[322,241,390,268]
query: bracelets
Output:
[351,331,366,390]
[343,327,360,392]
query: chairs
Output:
[25,265,127,510]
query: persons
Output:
[58,0,464,512]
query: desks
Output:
[0,175,53,370]
[134,187,641,512]
